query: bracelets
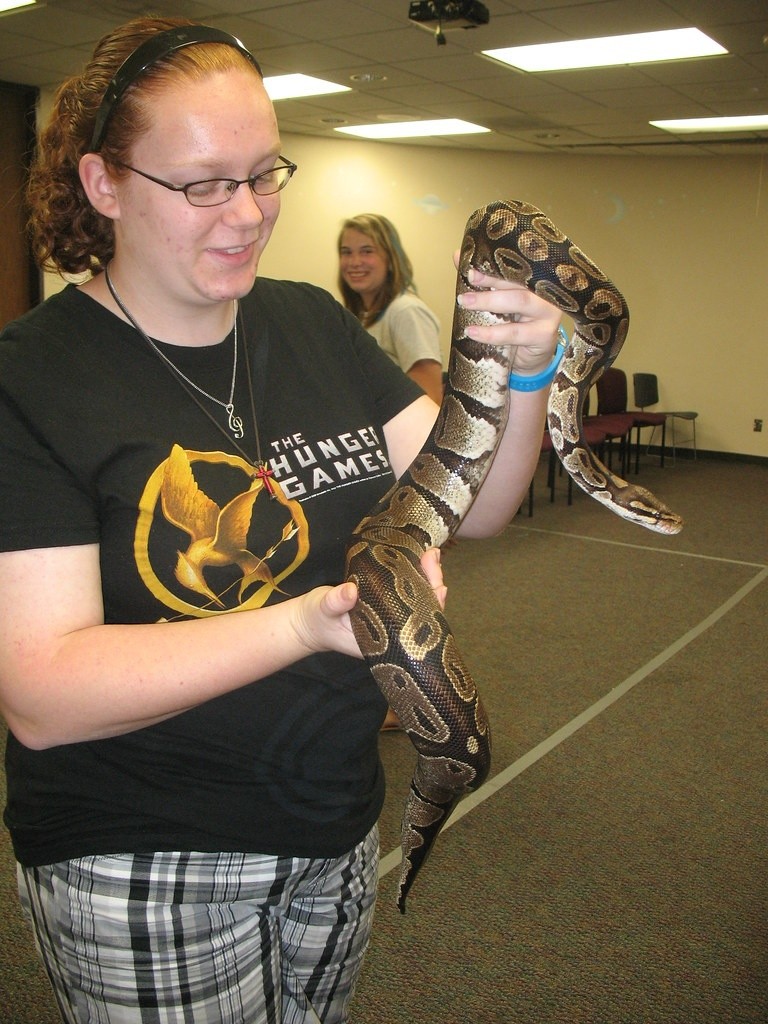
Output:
[511,323,568,391]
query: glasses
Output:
[96,152,297,207]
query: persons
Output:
[0,16,568,1024]
[338,214,444,407]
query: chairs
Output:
[516,364,698,517]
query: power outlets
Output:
[753,419,762,432]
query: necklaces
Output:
[104,256,278,500]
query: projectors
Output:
[409,0,490,49]
